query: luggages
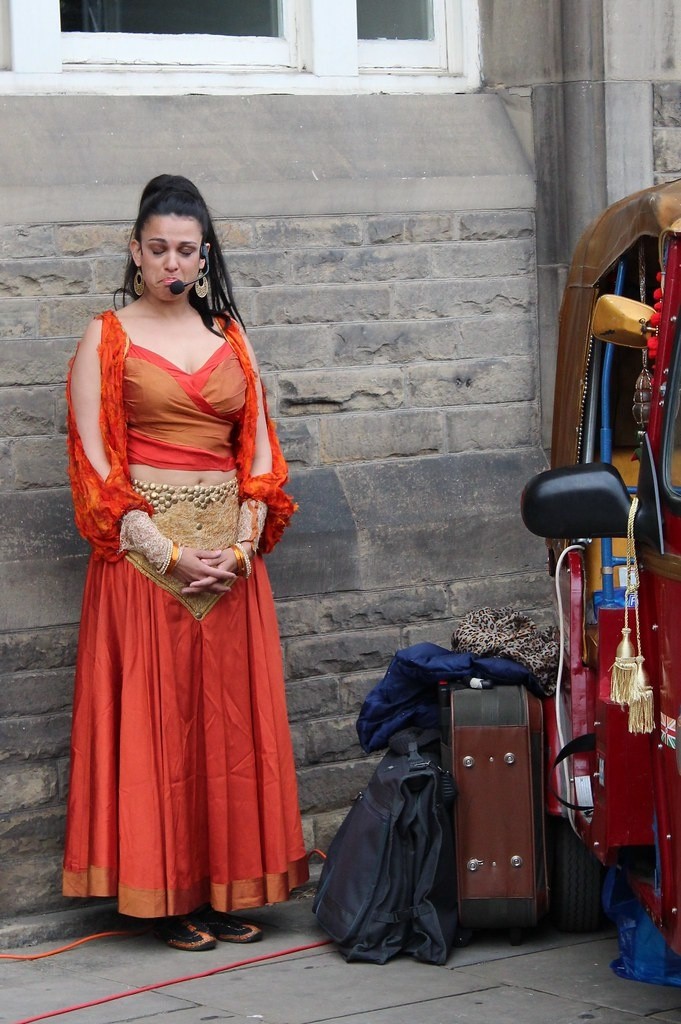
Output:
[450,684,549,945]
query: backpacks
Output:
[310,735,459,967]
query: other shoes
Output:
[197,909,262,943]
[153,919,216,950]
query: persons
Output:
[65,175,312,950]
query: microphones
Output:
[169,242,209,295]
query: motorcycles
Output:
[518,176,681,955]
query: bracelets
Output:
[157,540,183,575]
[231,543,252,579]
